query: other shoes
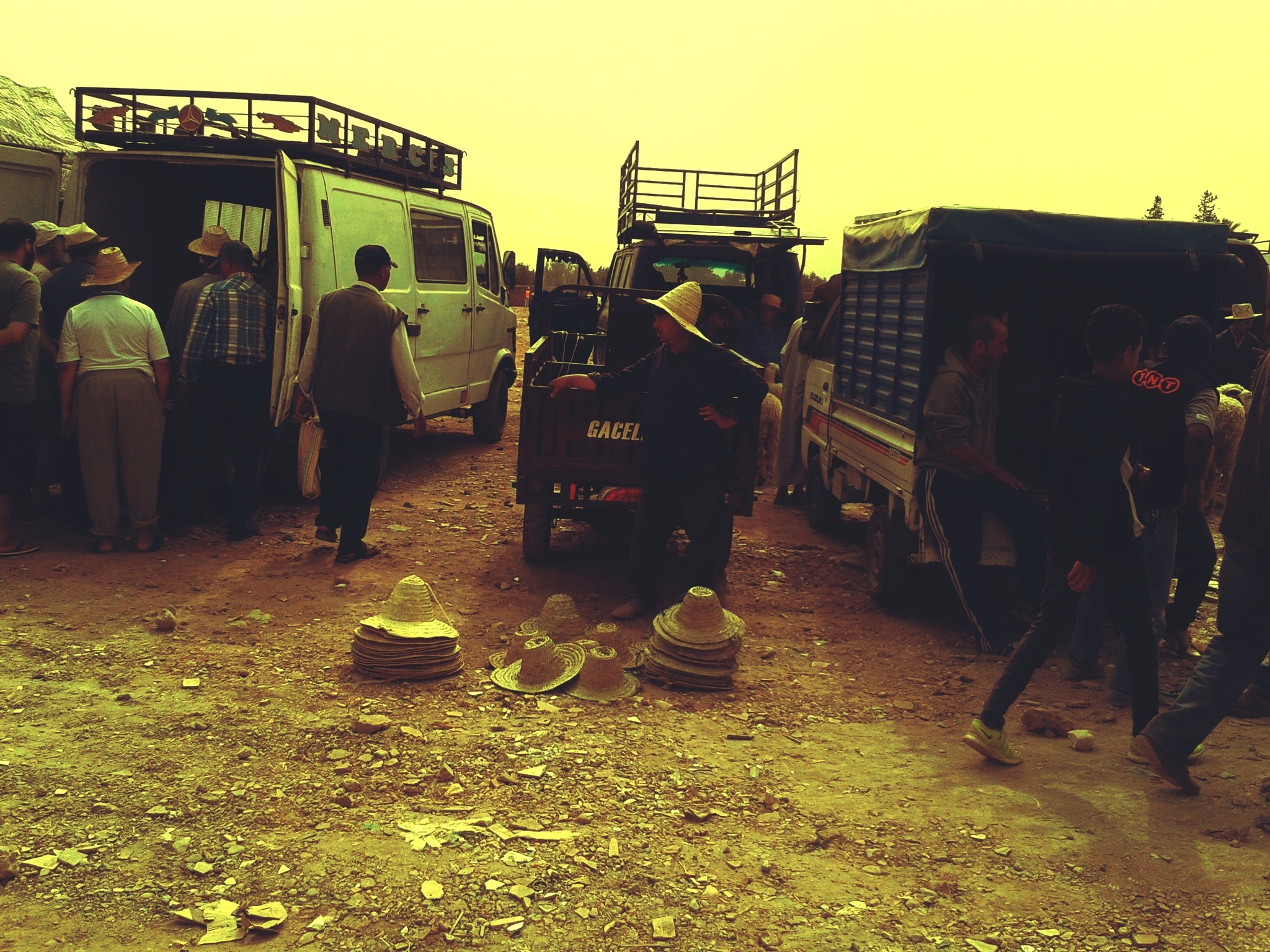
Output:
[1067,661,1107,681]
[1135,732,1201,794]
[610,593,653,619]
[1109,691,1167,706]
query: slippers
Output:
[134,529,166,552]
[0,535,41,556]
[92,533,115,554]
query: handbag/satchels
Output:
[298,417,325,499]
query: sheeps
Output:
[753,361,787,487]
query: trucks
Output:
[790,206,1270,614]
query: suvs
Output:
[1,141,519,484]
[533,239,804,391]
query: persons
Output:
[297,244,427,563]
[914,302,1270,794]
[699,301,729,340]
[743,293,791,368]
[0,217,276,556]
[774,309,814,506]
[549,280,768,619]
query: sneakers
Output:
[1128,733,1204,763]
[1228,680,1270,718]
[1161,616,1201,661]
[963,718,1024,767]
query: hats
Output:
[562,645,639,701]
[29,220,65,247]
[520,592,596,644]
[635,281,711,344]
[62,222,109,252]
[573,639,600,674]
[591,621,645,668]
[351,573,466,680]
[755,294,782,309]
[188,225,232,257]
[643,585,748,690]
[355,244,398,272]
[489,629,547,669]
[490,635,585,693]
[79,246,142,287]
[208,240,252,269]
[1225,303,1262,320]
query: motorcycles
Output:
[513,285,769,588]
